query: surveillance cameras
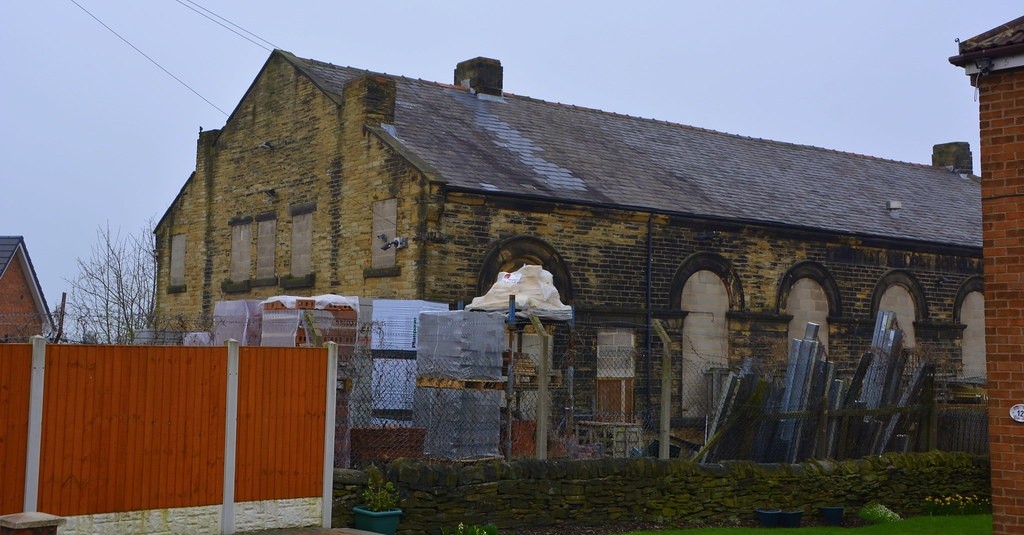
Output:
[381,242,392,251]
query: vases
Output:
[754,507,783,528]
[816,506,845,526]
[781,508,805,528]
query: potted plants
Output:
[352,477,403,535]
